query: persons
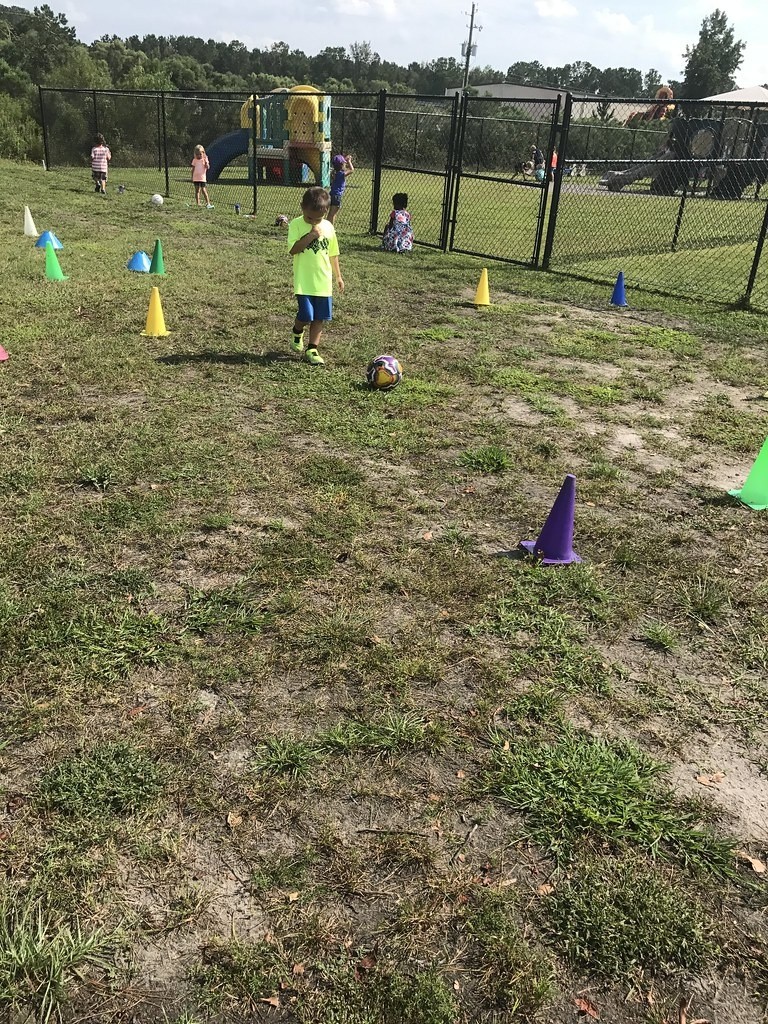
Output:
[90,133,112,195]
[511,145,558,184]
[328,154,356,226]
[287,186,345,365]
[380,193,415,253]
[190,144,210,208]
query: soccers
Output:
[275,214,288,225]
[366,354,403,391]
[151,194,163,204]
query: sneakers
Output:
[303,347,326,366]
[290,325,304,352]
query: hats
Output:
[331,154,348,168]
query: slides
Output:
[599,150,670,186]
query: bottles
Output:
[235,203,240,215]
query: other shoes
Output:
[95,183,101,193]
[100,189,106,194]
[206,204,215,209]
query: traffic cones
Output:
[42,242,69,282]
[728,432,768,511]
[148,239,167,276]
[610,271,628,306]
[21,205,39,236]
[140,287,171,337]
[468,268,494,306]
[519,473,584,565]
